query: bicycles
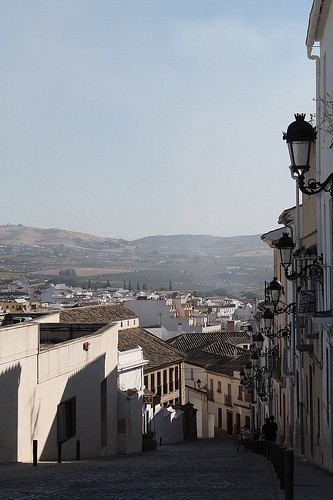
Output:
[235,432,246,452]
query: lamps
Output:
[274,232,324,296]
[263,277,296,321]
[261,308,291,349]
[196,379,207,391]
[237,333,280,404]
[282,112,333,200]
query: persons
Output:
[260,415,277,444]
[252,428,261,442]
[239,424,249,453]
